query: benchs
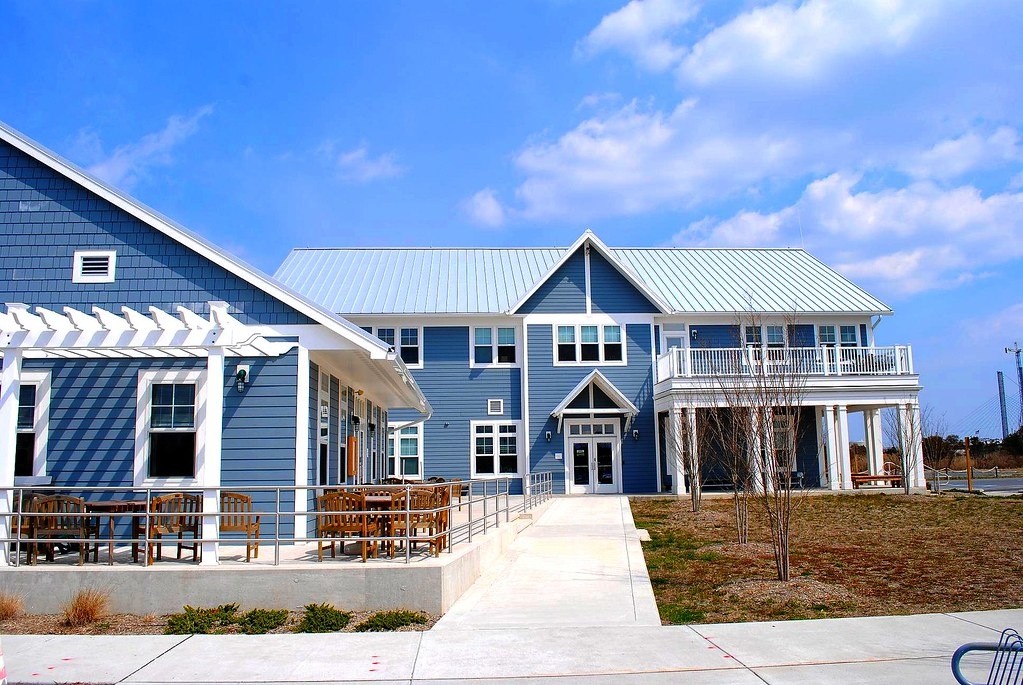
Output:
[851,475,903,489]
[701,479,735,486]
[776,477,805,489]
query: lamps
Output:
[546,431,552,442]
[351,412,360,433]
[633,429,640,441]
[236,364,249,393]
[906,403,912,411]
[692,329,698,340]
[368,419,375,437]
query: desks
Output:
[85,500,162,563]
[352,490,390,495]
[334,496,416,556]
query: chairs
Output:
[317,476,462,563]
[200,493,260,563]
[13,493,97,566]
[139,493,199,565]
